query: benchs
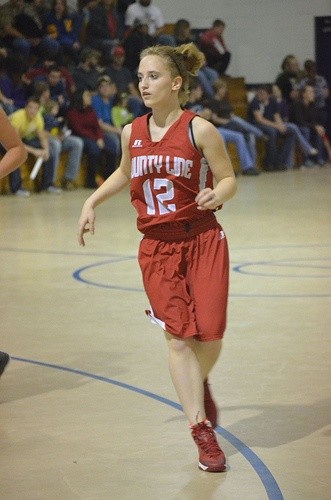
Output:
[0,76,304,195]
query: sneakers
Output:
[202,377,219,429]
[192,421,228,473]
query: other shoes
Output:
[63,180,86,191]
[39,186,63,195]
[14,189,31,198]
[307,147,321,156]
[243,168,263,177]
[85,177,100,190]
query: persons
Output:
[0,0,331,198]
[78,42,237,473]
